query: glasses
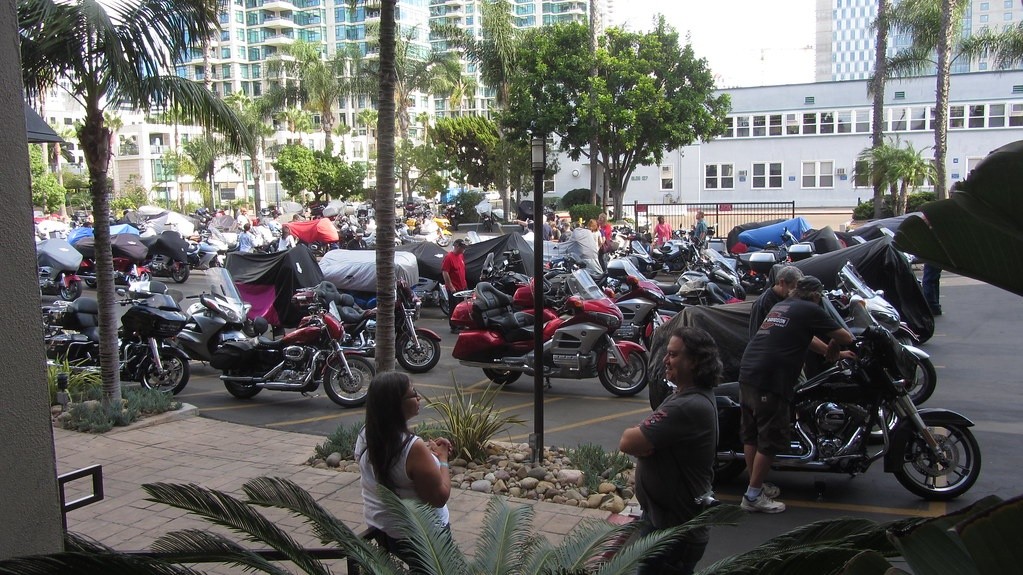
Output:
[403,387,418,400]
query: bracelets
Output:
[440,462,450,468]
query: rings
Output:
[454,289,455,290]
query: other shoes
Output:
[931,304,942,316]
[450,328,464,334]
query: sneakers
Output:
[760,483,781,500]
[741,488,786,513]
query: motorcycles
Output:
[34,188,802,412]
[659,323,985,504]
[650,212,941,449]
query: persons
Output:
[236,201,367,254]
[439,239,470,333]
[620,326,723,575]
[37,208,131,238]
[652,215,672,250]
[738,266,855,513]
[514,212,612,278]
[354,371,452,575]
[695,211,709,259]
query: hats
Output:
[455,239,467,248]
